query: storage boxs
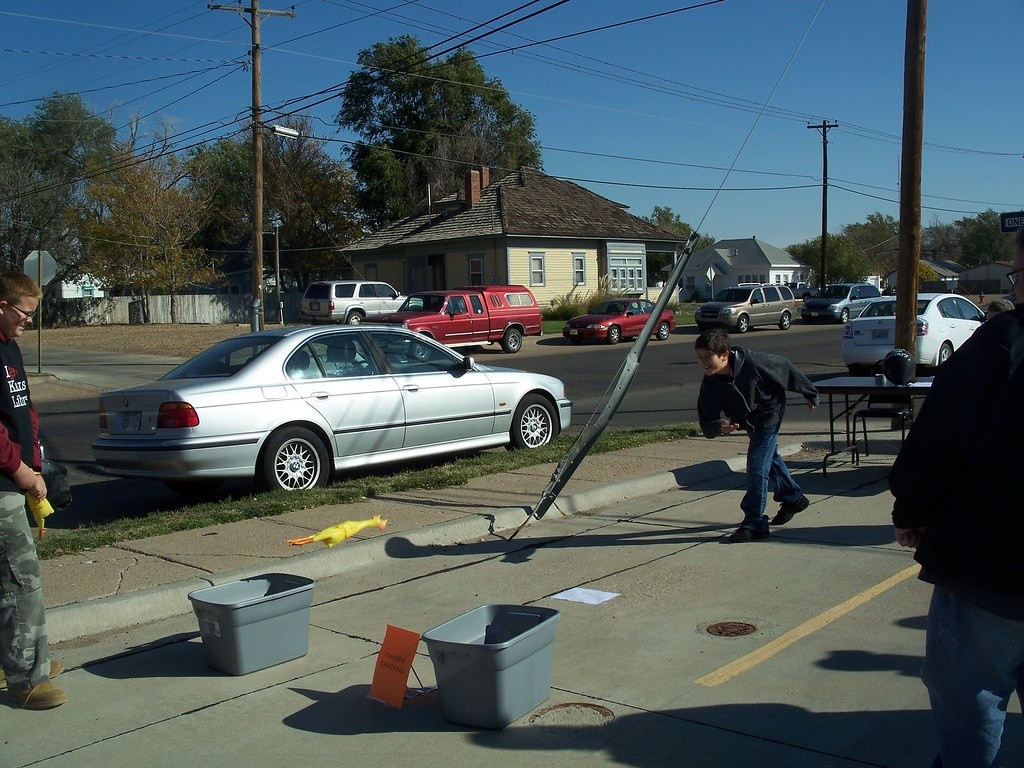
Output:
[421,603,561,731]
[187,572,317,677]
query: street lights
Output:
[271,221,283,324]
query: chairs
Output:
[852,358,914,464]
[284,347,310,374]
[428,301,442,313]
[408,305,423,312]
[325,338,366,377]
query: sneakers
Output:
[770,495,809,525]
[728,515,770,542]
[0,659,64,688]
[7,679,66,710]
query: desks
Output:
[809,377,935,478]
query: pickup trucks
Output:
[358,284,543,354]
[784,281,820,303]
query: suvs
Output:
[297,279,424,327]
[693,281,797,332]
[800,283,881,325]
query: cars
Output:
[842,293,988,378]
[90,324,565,497]
[560,296,677,343]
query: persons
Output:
[988,298,1015,320]
[882,286,893,296]
[0,270,67,710]
[694,328,821,543]
[888,225,1024,768]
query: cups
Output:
[875,373,886,384]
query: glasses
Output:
[1007,268,1024,285]
[7,302,37,318]
